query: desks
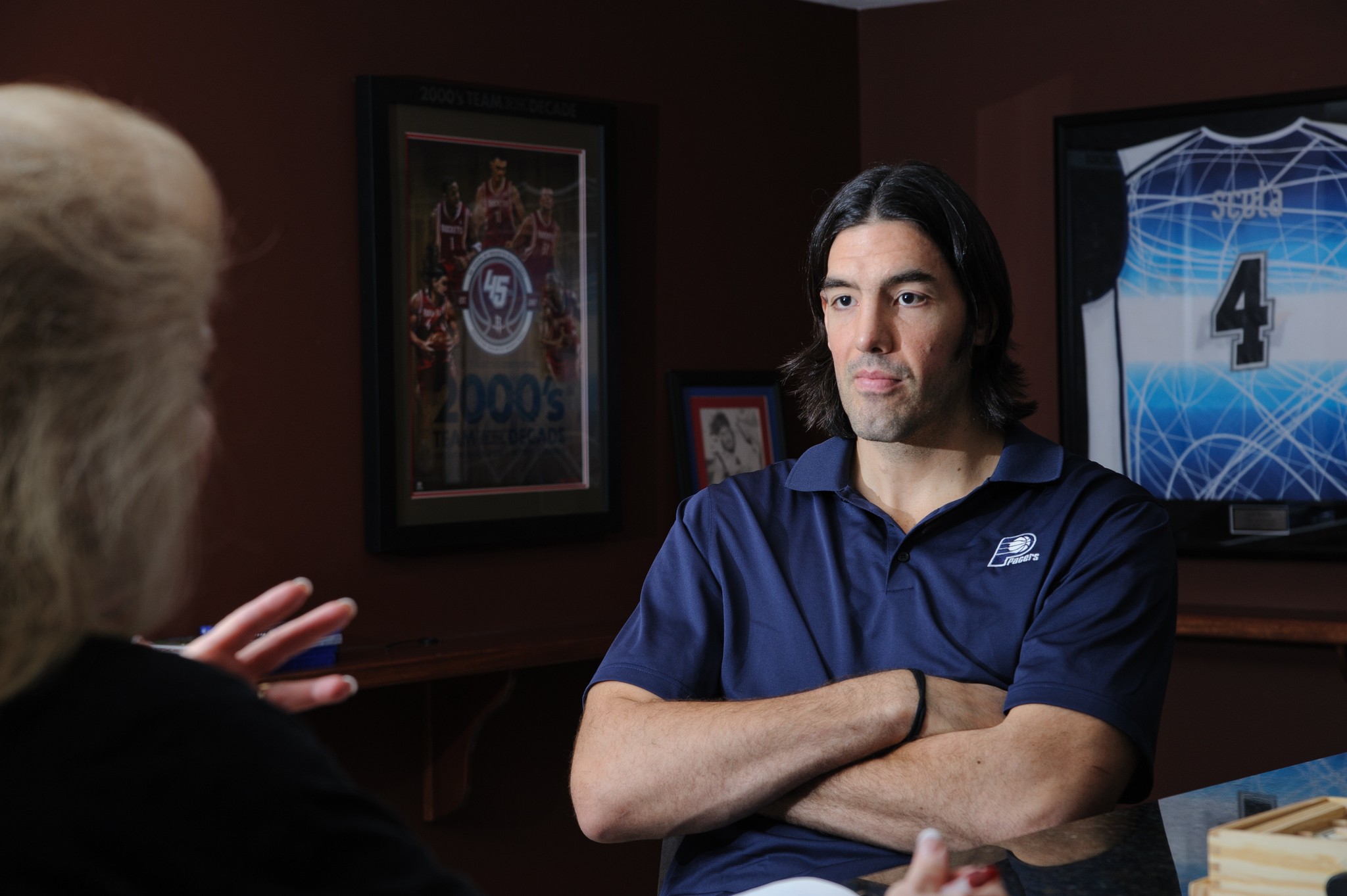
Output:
[833,745,1347,896]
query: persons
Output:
[409,154,577,480]
[568,163,1180,895]
[0,81,998,896]
[708,412,762,484]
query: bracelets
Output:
[900,668,926,746]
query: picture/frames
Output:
[349,69,633,560]
[666,363,813,504]
